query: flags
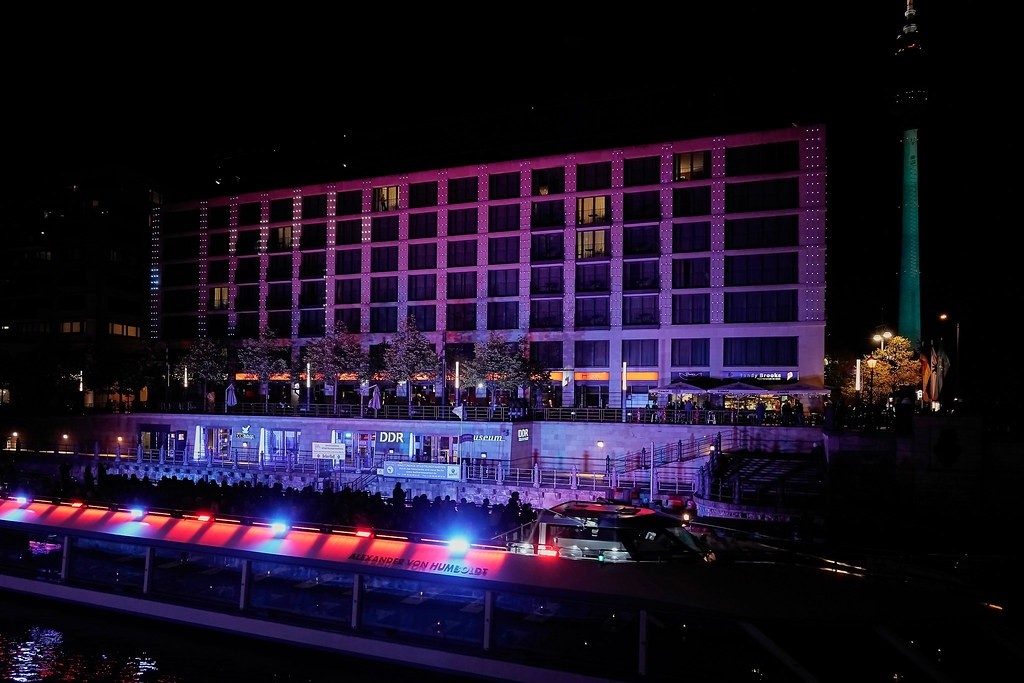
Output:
[919,347,950,402]
[452,406,463,419]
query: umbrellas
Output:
[648,382,707,423]
[707,382,831,423]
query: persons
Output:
[685,396,712,425]
[652,400,673,409]
[782,400,804,427]
[421,452,429,463]
[82,461,538,541]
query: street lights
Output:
[939,314,946,350]
[855,358,861,401]
[306,361,311,413]
[454,361,461,410]
[184,364,188,413]
[623,361,626,423]
[873,332,893,352]
[79,369,83,414]
[866,354,878,405]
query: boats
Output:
[0,492,944,683]
